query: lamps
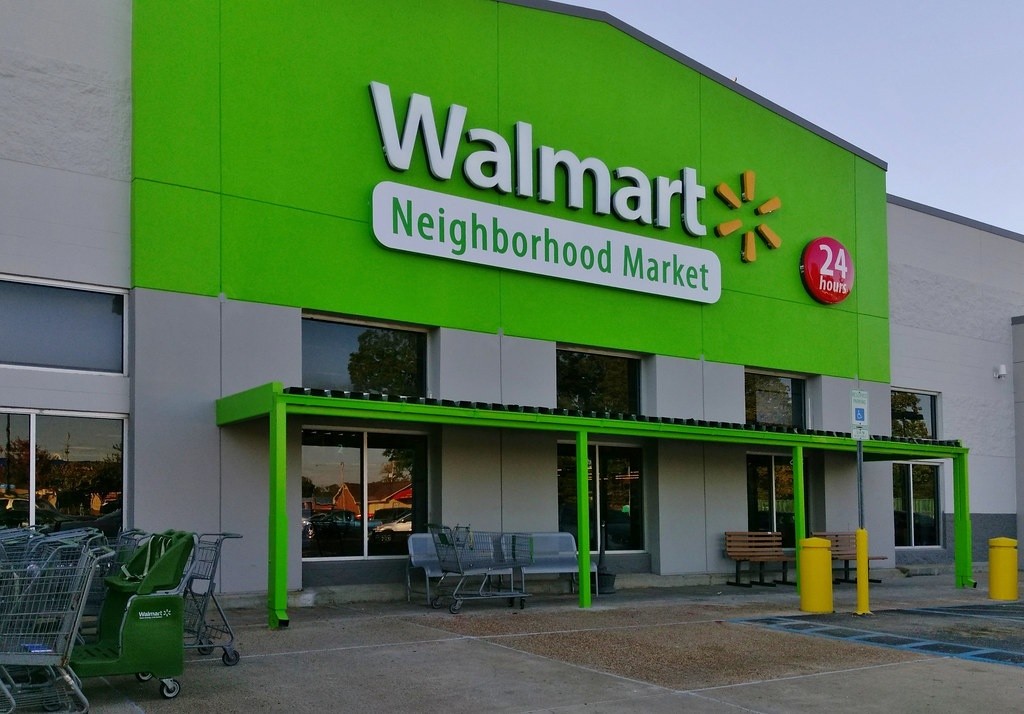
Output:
[994,364,1008,378]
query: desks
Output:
[812,532,888,584]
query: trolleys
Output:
[0,524,238,714]
[423,524,533,613]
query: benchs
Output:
[724,531,797,587]
[406,532,599,604]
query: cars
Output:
[302,509,413,544]
[0,495,121,537]
